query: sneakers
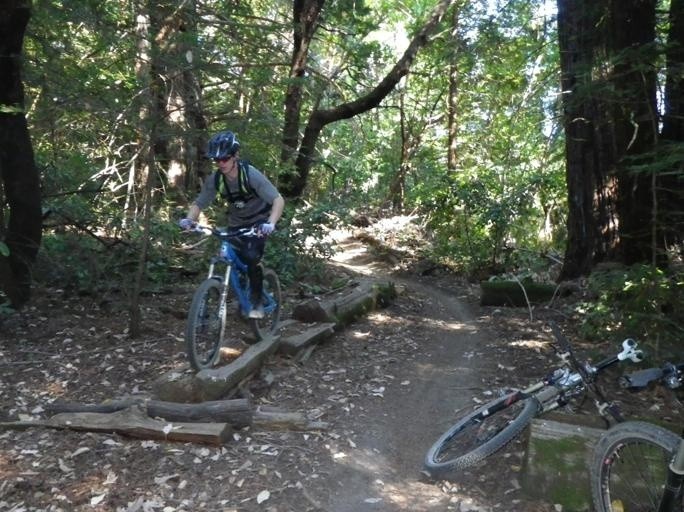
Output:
[239,272,249,290]
[249,302,266,319]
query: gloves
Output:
[179,219,194,230]
[256,224,273,237]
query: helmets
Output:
[208,131,239,159]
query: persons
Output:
[175,128,285,322]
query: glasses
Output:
[212,154,233,163]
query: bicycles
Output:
[423,325,684,511]
[178,221,285,372]
[620,362,683,511]
